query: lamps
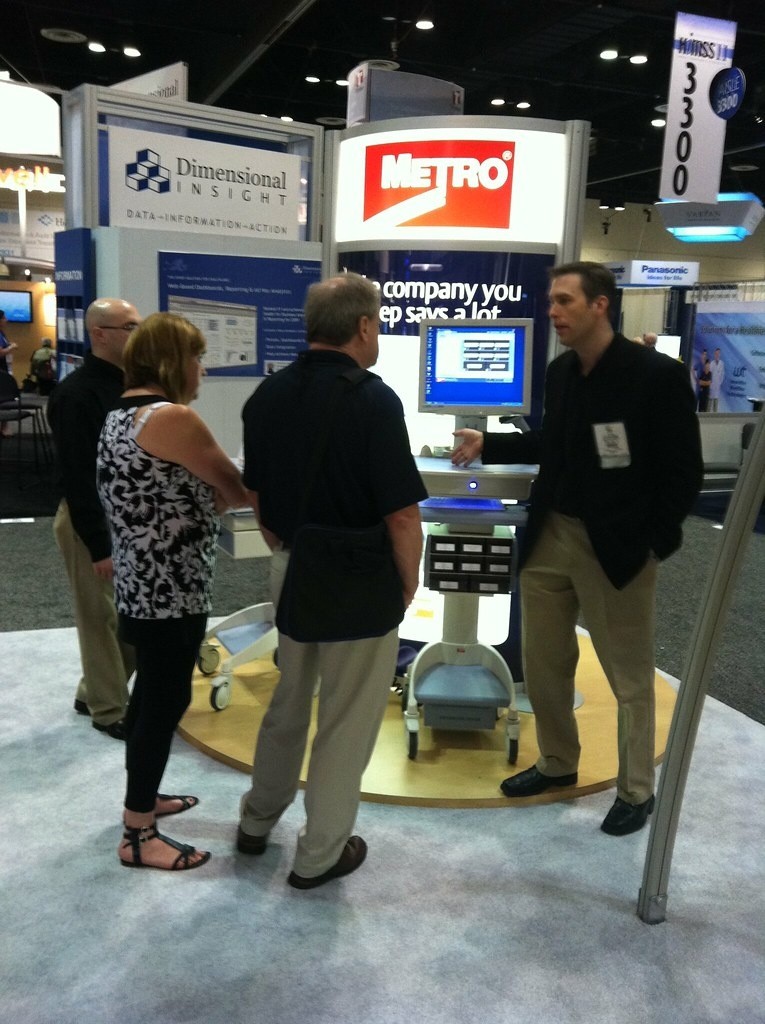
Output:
[595,178,626,211]
[39,25,88,43]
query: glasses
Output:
[99,323,139,335]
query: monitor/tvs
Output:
[654,334,682,359]
[0,290,33,322]
[417,316,534,450]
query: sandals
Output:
[120,820,211,870]
[155,793,199,817]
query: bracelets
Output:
[8,346,11,349]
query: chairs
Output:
[0,359,62,492]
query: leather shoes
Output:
[74,698,90,715]
[236,823,266,855]
[601,793,655,837]
[288,836,367,889]
[499,764,577,798]
[92,718,126,740]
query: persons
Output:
[30,337,53,395]
[237,271,429,891]
[0,309,18,432]
[47,298,143,741]
[630,333,657,350]
[93,311,246,870]
[448,260,706,835]
[692,348,724,414]
